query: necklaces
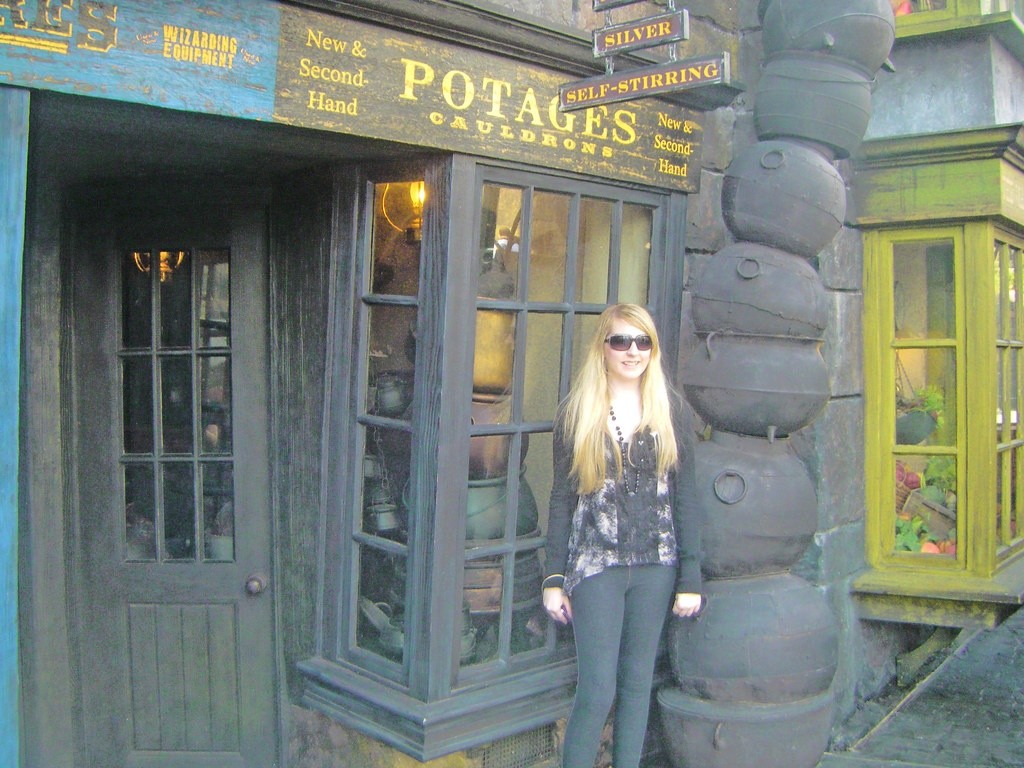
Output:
[609,406,640,497]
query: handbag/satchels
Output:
[896,410,938,446]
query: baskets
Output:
[896,479,912,512]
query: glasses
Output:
[603,335,653,351]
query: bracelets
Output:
[541,574,564,593]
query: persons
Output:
[543,304,702,768]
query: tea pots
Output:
[377,373,409,417]
[365,492,400,535]
